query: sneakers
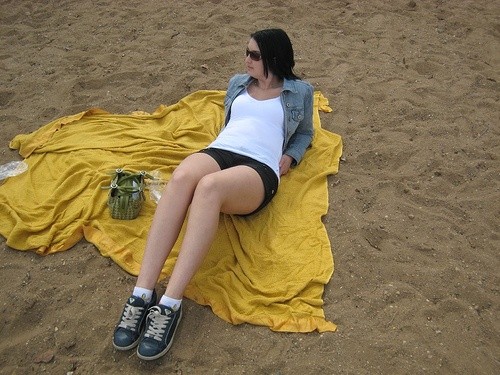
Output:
[112,287,158,351]
[136,301,183,361]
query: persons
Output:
[112,25,314,361]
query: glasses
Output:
[246,47,262,62]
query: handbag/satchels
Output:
[107,167,155,219]
